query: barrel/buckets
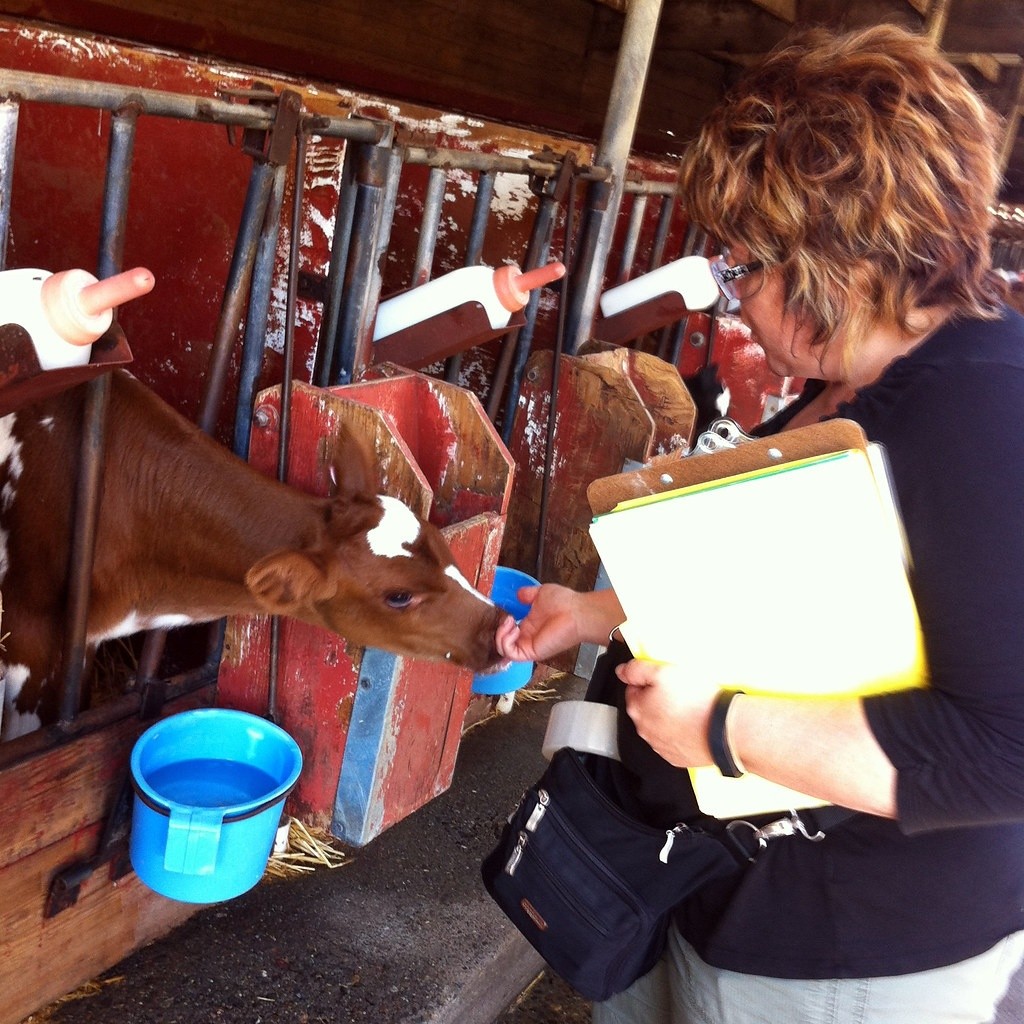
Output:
[129,708,302,902]
[472,566,541,694]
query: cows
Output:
[2,365,512,743]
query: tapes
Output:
[541,700,619,761]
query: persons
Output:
[495,22,1023,1024]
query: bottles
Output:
[600,255,735,319]
[372,261,566,342]
[0,267,155,371]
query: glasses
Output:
[712,247,787,302]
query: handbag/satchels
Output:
[482,743,763,1003]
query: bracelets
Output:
[705,686,746,778]
